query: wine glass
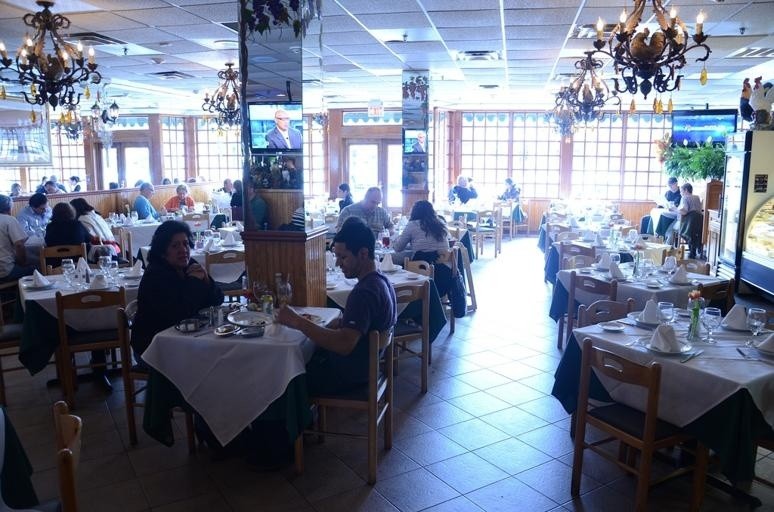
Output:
[382,229,390,253]
[326,253,336,279]
[253,281,267,308]
[276,282,293,311]
[103,256,111,279]
[108,212,116,224]
[106,261,119,284]
[132,211,138,225]
[99,257,106,278]
[687,297,705,328]
[111,214,118,226]
[745,307,768,347]
[63,264,76,288]
[656,301,674,324]
[211,231,220,245]
[609,253,620,266]
[702,307,723,344]
[204,228,213,242]
[663,256,676,280]
[628,229,637,242]
[62,258,73,282]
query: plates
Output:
[639,337,692,354]
[214,324,241,336]
[405,273,422,279]
[627,310,677,325]
[381,264,402,272]
[602,273,628,282]
[22,280,56,289]
[227,310,273,327]
[597,321,625,331]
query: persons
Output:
[403,76,428,102]
[411,131,426,153]
[132,219,252,459]
[0,176,122,280]
[654,177,683,241]
[218,178,242,209]
[338,184,353,212]
[394,200,451,260]
[502,178,526,221]
[337,186,395,238]
[667,182,703,259]
[262,216,398,474]
[252,110,303,189]
[449,176,478,228]
[134,178,196,220]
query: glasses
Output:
[277,117,290,121]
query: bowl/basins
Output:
[180,319,199,332]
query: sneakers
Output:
[687,252,696,259]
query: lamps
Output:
[0,0,120,127]
[201,62,242,136]
[587,0,710,114]
[550,51,623,141]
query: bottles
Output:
[77,269,82,286]
[85,267,90,283]
[210,305,223,327]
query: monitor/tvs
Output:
[247,100,302,156]
[402,128,429,154]
[672,109,737,148]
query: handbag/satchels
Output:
[210,215,228,231]
